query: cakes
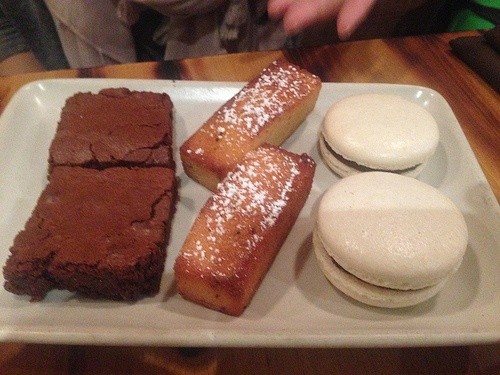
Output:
[4,164,180,303]
[48,86,172,180]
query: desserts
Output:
[318,93,442,178]
[312,172,471,308]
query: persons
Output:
[43,0,375,69]
[0,0,70,76]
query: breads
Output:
[173,141,316,318]
[180,56,323,193]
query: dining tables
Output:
[0,30,500,375]
